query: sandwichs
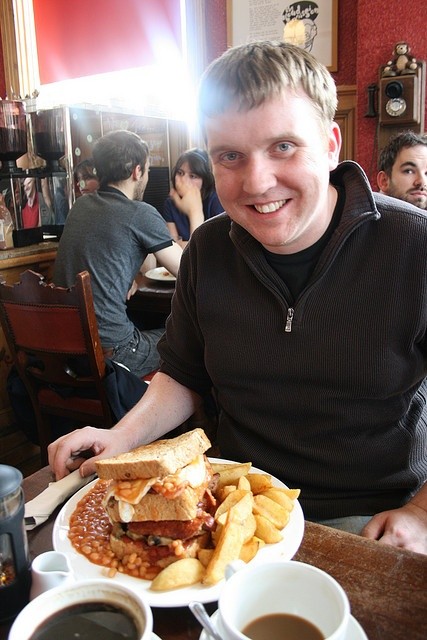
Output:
[95,428,211,564]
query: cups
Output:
[217,559,350,640]
[8,581,151,640]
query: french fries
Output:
[147,461,301,593]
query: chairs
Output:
[1,269,113,440]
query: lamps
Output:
[283,18,306,47]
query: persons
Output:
[164,146,227,248]
[377,131,426,211]
[20,176,41,226]
[44,39,427,555]
[49,127,207,373]
[73,158,99,193]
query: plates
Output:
[200,611,368,640]
[146,267,175,282]
[52,458,305,607]
